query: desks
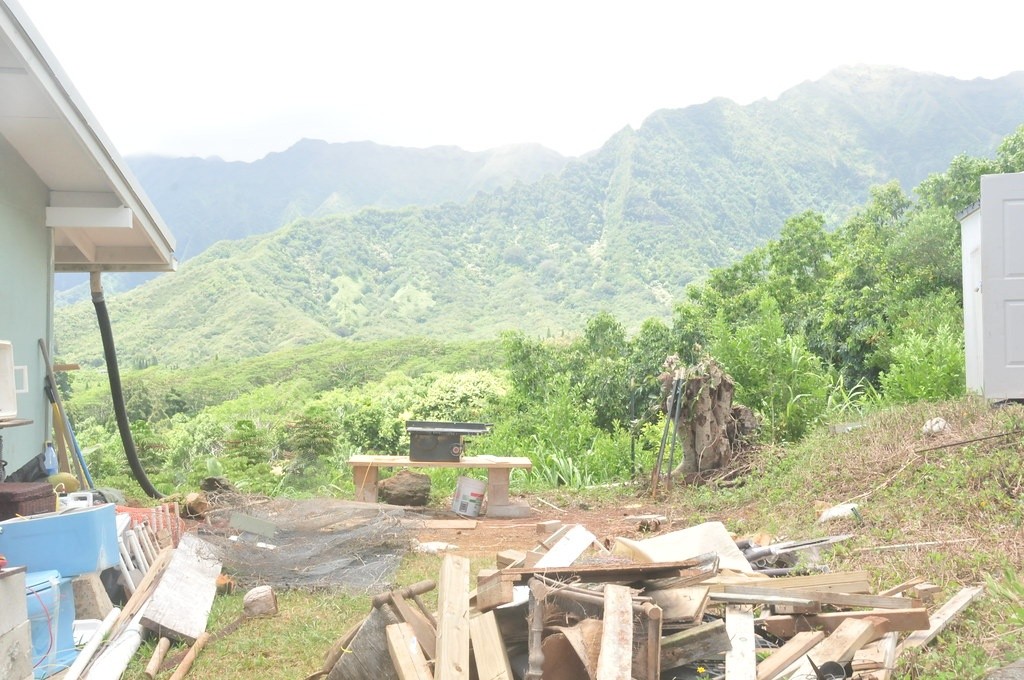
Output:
[0,419,34,482]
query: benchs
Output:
[350,455,531,508]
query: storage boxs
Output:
[410,432,463,463]
[0,503,119,576]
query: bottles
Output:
[44,442,58,475]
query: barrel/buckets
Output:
[452,476,488,519]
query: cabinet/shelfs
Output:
[0,566,34,680]
